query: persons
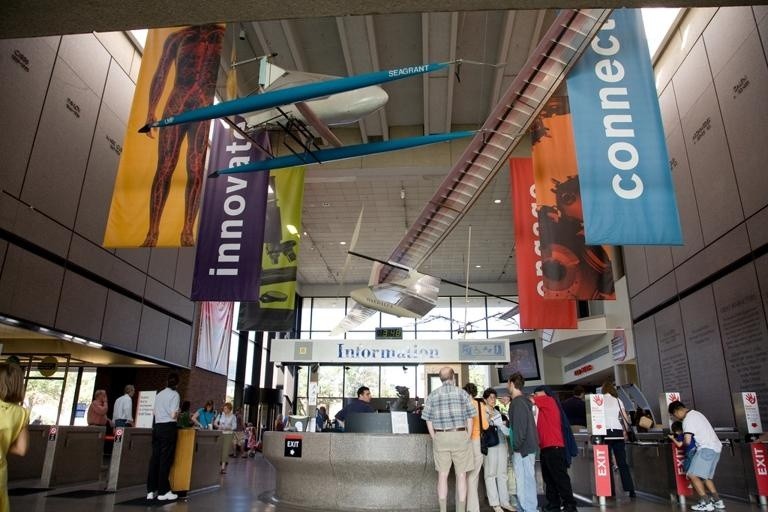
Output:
[459,372,727,512]
[141,23,225,247]
[88,387,112,465]
[232,408,244,458]
[243,422,249,452]
[0,360,31,512]
[333,386,377,428]
[316,405,332,433]
[274,412,284,430]
[243,420,257,456]
[177,399,194,429]
[192,400,216,431]
[110,384,139,428]
[422,366,479,510]
[216,403,238,477]
[147,373,181,501]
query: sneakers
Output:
[691,497,726,511]
[157,491,178,500]
[147,491,158,500]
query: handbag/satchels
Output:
[626,428,637,441]
[480,430,488,455]
[488,424,499,447]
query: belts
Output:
[434,428,466,432]
